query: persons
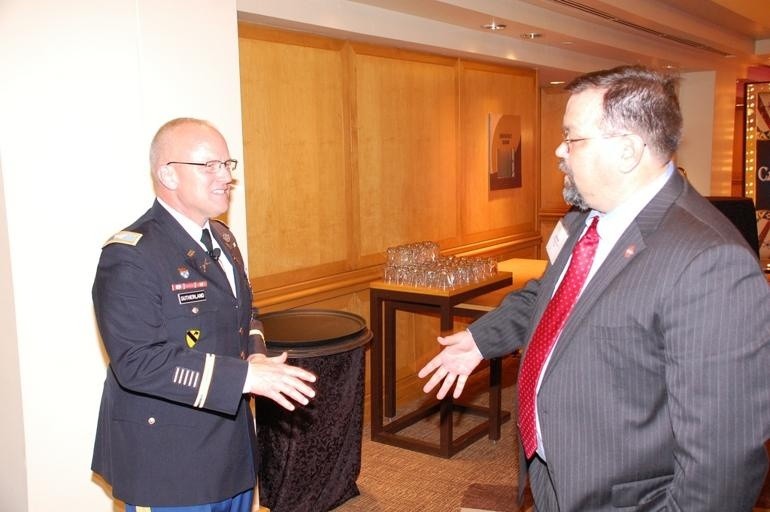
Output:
[91,118,317,512]
[418,65,770,512]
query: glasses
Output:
[560,134,622,156]
[167,160,237,174]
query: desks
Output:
[367,270,515,460]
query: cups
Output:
[384,242,499,288]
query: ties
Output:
[518,217,600,461]
[201,228,220,264]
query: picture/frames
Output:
[741,80,770,275]
